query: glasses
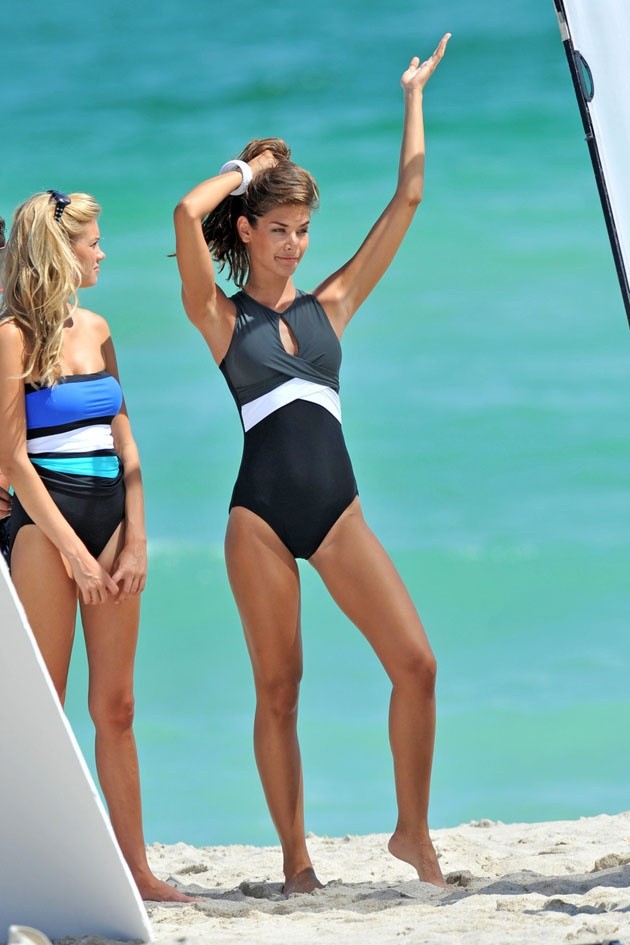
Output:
[47,189,71,221]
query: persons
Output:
[0,217,14,555]
[0,193,193,901]
[174,32,452,896]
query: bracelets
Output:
[219,160,252,196]
